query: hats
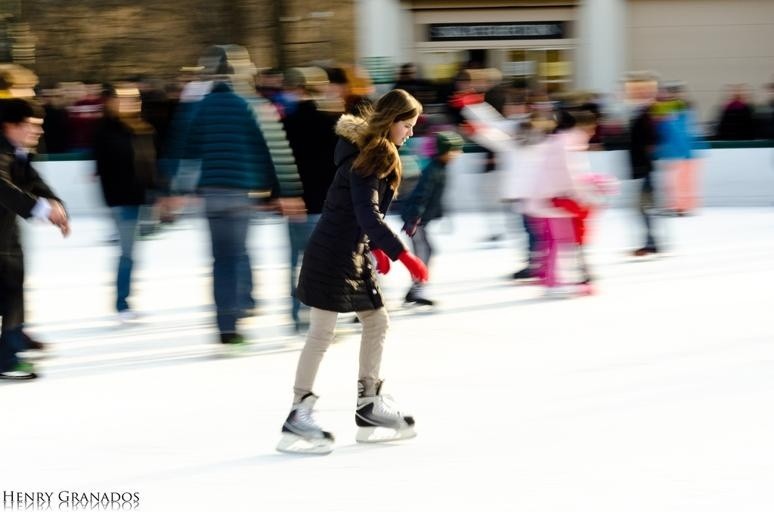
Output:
[434,129,464,155]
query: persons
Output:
[272,88,430,454]
[0,39,771,385]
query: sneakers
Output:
[282,393,337,443]
[513,265,545,280]
[406,286,434,306]
[1,320,46,380]
[355,378,417,430]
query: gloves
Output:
[371,248,392,274]
[402,216,422,236]
[398,251,429,282]
